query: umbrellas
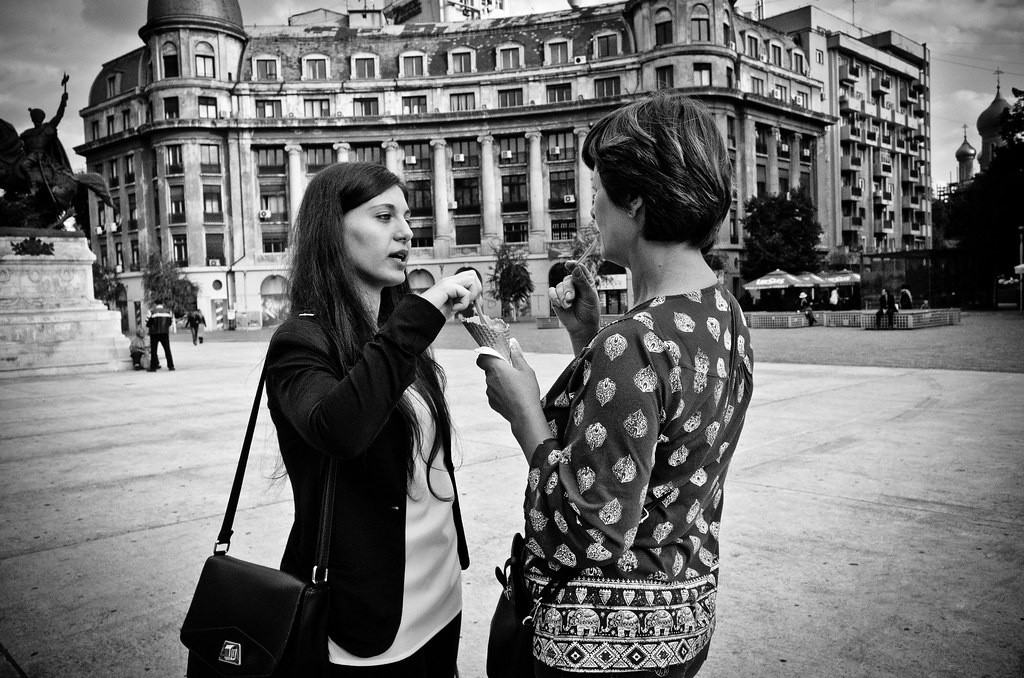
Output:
[742,268,861,290]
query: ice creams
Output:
[461,315,511,362]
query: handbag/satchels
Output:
[179,551,327,677]
[894,304,899,312]
[486,532,536,678]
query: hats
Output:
[799,292,807,299]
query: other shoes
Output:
[148,369,156,371]
[135,366,140,370]
[169,368,175,370]
[157,366,161,368]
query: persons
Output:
[185,309,201,345]
[129,328,161,370]
[797,292,817,327]
[475,96,754,678]
[900,284,912,309]
[921,300,930,309]
[20,93,68,198]
[266,162,483,678]
[830,289,839,312]
[145,299,176,372]
[875,288,897,330]
[197,310,206,343]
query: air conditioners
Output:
[781,144,788,151]
[803,149,809,156]
[794,96,802,106]
[107,219,122,234]
[759,54,767,63]
[206,56,589,268]
[727,40,736,51]
[771,89,780,99]
[93,227,106,235]
